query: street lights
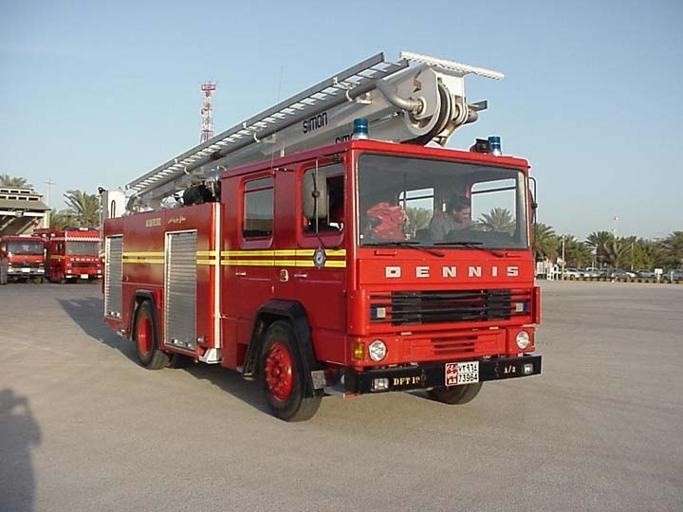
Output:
[45,178,57,233]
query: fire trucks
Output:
[94,46,545,426]
[32,224,105,286]
[1,234,47,287]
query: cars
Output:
[552,264,682,281]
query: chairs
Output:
[416,228,428,242]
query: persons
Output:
[429,195,483,237]
